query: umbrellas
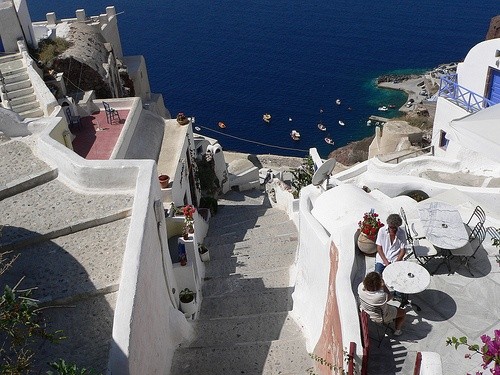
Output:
[445,103,500,164]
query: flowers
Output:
[446,328,500,375]
[184,205,195,226]
[177,112,187,121]
[179,287,194,302]
[357,209,386,240]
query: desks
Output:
[381,261,430,309]
[420,202,469,274]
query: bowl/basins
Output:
[408,273,413,278]
[442,223,447,228]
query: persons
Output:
[374,214,407,272]
[357,272,406,335]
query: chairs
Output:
[400,207,437,267]
[437,206,487,278]
[103,102,121,124]
[66,107,81,127]
[359,297,397,347]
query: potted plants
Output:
[183,230,188,239]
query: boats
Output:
[317,124,327,131]
[218,122,226,129]
[263,113,272,123]
[324,137,335,145]
[377,105,397,112]
[338,120,344,126]
[289,130,300,141]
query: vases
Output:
[178,118,189,125]
[198,246,210,262]
[180,299,197,316]
[159,175,170,188]
[189,225,194,233]
[358,232,377,254]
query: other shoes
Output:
[394,329,402,335]
